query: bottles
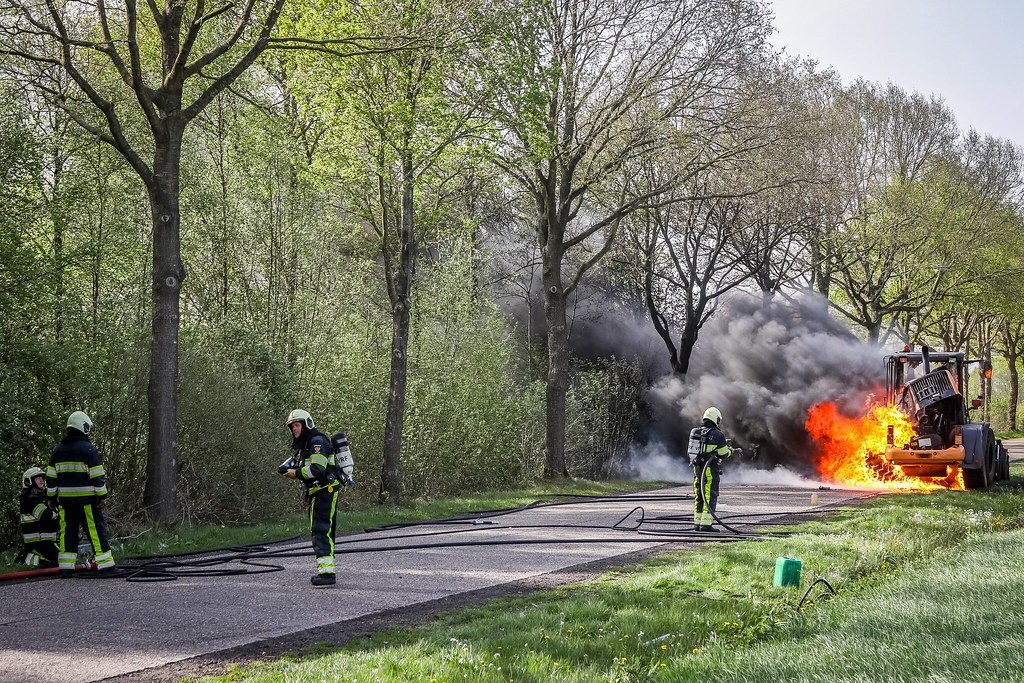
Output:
[811,493,818,505]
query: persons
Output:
[14,467,60,569]
[47,411,124,574]
[283,409,341,587]
[687,407,733,533]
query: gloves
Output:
[726,439,732,447]
[47,499,58,511]
[731,447,743,458]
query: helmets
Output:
[67,411,93,436]
[22,467,46,489]
[703,407,723,428]
[286,409,316,430]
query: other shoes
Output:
[699,525,720,533]
[310,573,336,586]
[694,524,700,531]
[60,571,73,579]
[99,568,125,574]
[14,549,30,566]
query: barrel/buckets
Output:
[774,557,801,589]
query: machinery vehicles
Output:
[868,337,1013,491]
